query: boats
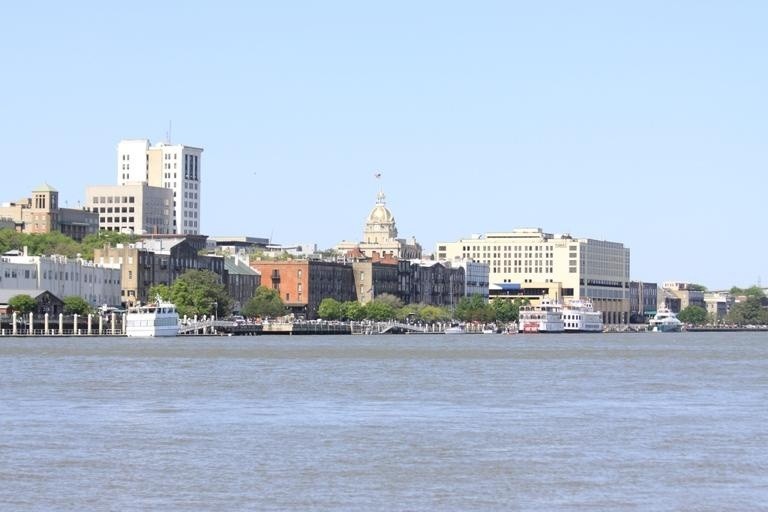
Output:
[123,296,180,338]
[558,299,605,333]
[648,303,681,333]
[518,299,566,333]
